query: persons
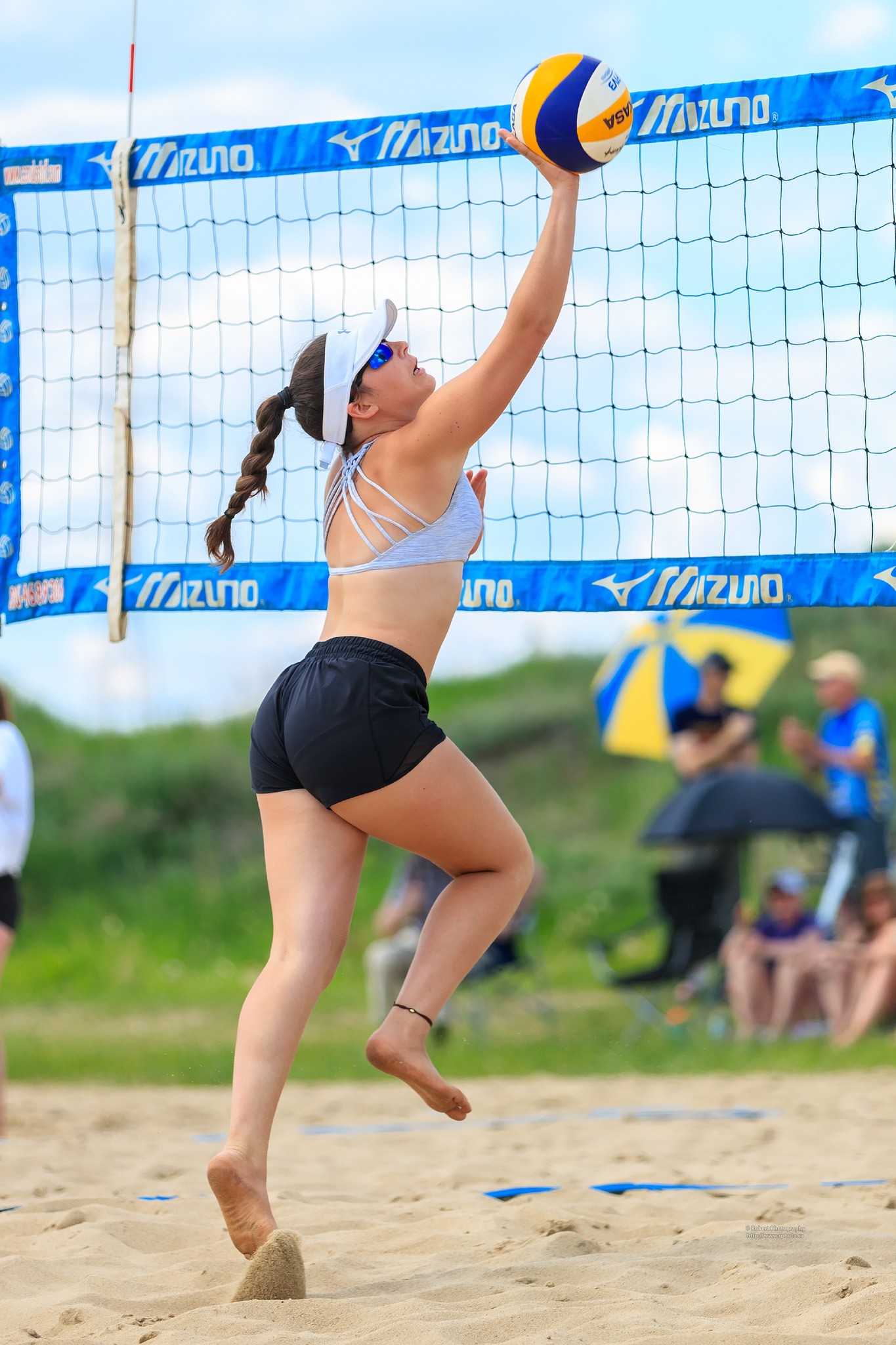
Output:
[205,128,580,1258]
[366,853,541,1025]
[717,865,835,1042]
[666,653,761,787]
[777,648,896,929]
[0,677,34,964]
[817,868,896,1048]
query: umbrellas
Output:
[591,607,795,762]
[642,765,843,842]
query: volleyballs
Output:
[510,53,633,174]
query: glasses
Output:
[356,339,393,388]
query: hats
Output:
[767,866,807,895]
[319,300,398,468]
